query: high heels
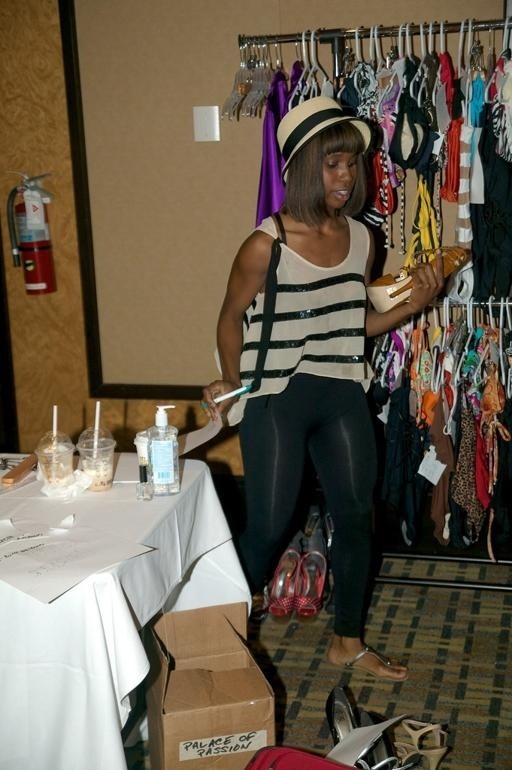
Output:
[395,719,448,770]
[365,246,466,314]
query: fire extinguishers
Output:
[7,173,58,296]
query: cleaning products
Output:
[146,406,179,497]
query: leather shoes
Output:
[358,711,392,770]
[324,685,356,746]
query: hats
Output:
[277,97,373,183]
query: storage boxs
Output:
[146,600,276,770]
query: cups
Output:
[32,430,76,498]
[134,430,154,487]
[75,426,118,492]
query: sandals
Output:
[269,547,322,622]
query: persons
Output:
[195,93,448,680]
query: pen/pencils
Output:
[198,384,254,410]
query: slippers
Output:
[327,648,409,684]
